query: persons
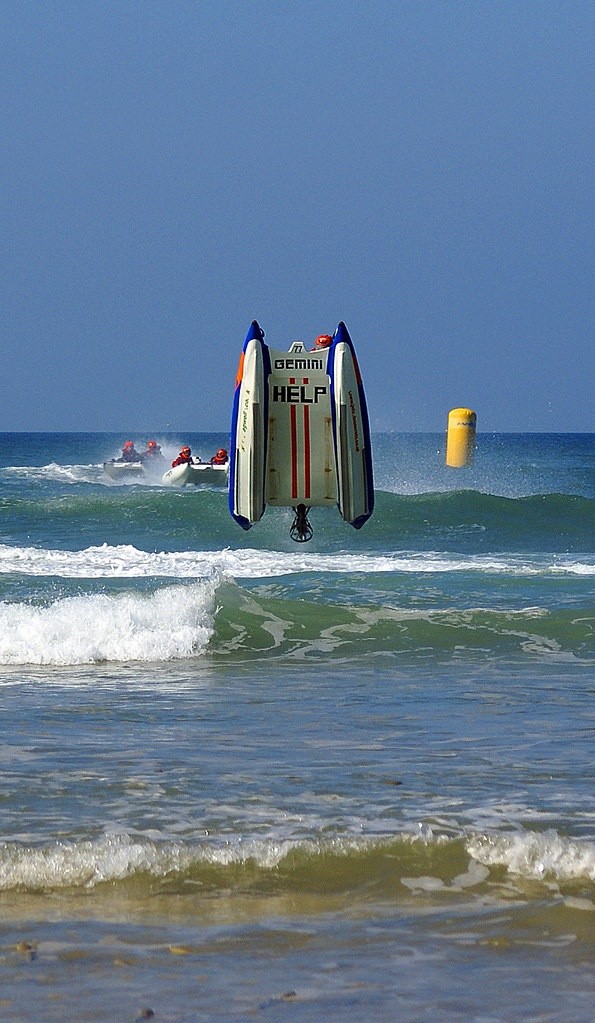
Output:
[172,447,194,467]
[211,449,229,464]
[122,441,166,461]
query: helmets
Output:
[316,334,332,346]
[182,447,191,455]
[126,442,133,446]
[148,442,155,448]
[217,449,226,457]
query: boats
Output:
[162,462,228,492]
[228,321,374,542]
[104,461,164,479]
